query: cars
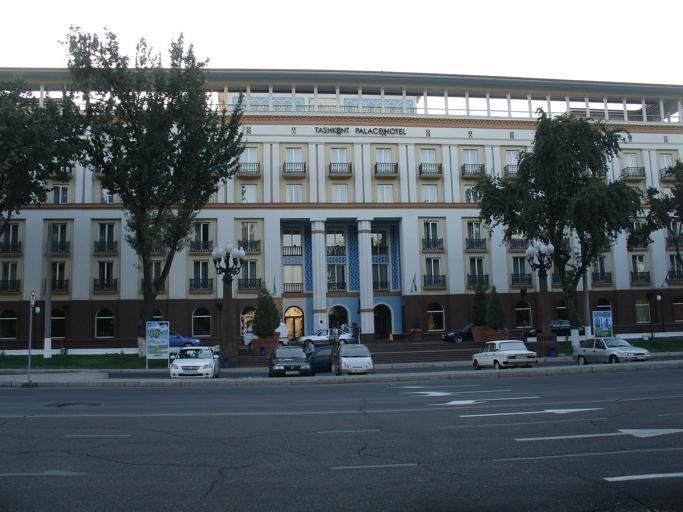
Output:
[338,344,376,375]
[442,323,497,343]
[169,329,201,347]
[307,345,338,372]
[472,339,537,369]
[571,337,651,363]
[170,345,220,378]
[297,328,355,346]
[528,320,585,336]
[265,345,315,376]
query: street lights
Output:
[212,242,245,368]
[525,236,560,356]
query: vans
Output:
[243,323,288,349]
